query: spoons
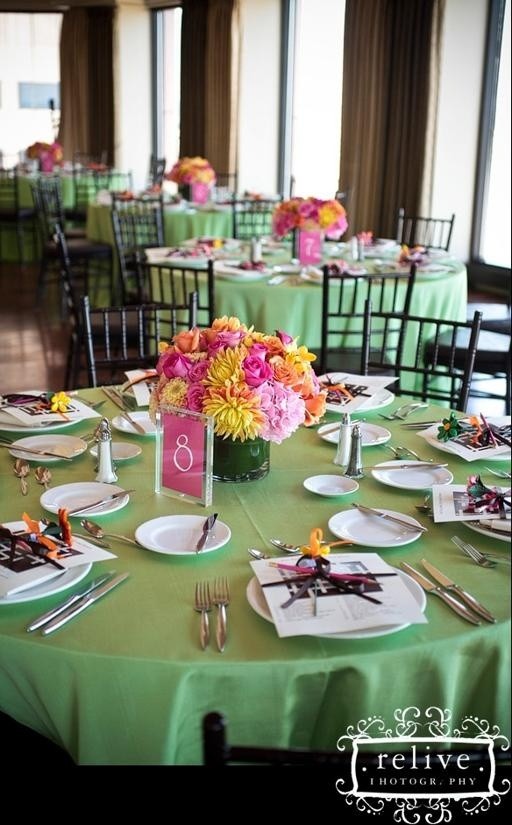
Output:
[249,537,325,561]
[9,458,51,496]
[380,443,432,461]
[79,520,149,550]
[412,493,431,512]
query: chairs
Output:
[1,128,511,825]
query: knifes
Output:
[38,570,129,638]
[399,558,484,627]
[319,418,366,436]
[119,411,147,435]
[59,488,139,517]
[400,421,435,428]
[22,571,114,635]
[360,462,448,471]
[193,513,220,553]
[419,555,500,625]
[0,441,77,462]
[351,504,427,533]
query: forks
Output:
[484,466,511,480]
[69,394,107,410]
[376,400,431,420]
[450,535,511,570]
[212,574,231,653]
[196,580,215,648]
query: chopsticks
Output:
[101,386,134,410]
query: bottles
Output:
[346,425,363,480]
[92,417,119,483]
[331,413,351,468]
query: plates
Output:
[133,515,232,557]
[245,564,428,640]
[116,193,250,215]
[328,507,423,549]
[38,482,131,518]
[366,460,453,490]
[111,411,162,436]
[461,520,512,544]
[298,474,360,498]
[424,438,511,462]
[0,562,95,606]
[316,420,391,447]
[8,434,89,463]
[0,420,84,433]
[328,388,395,414]
[151,234,451,288]
[122,381,133,398]
[90,442,143,462]
